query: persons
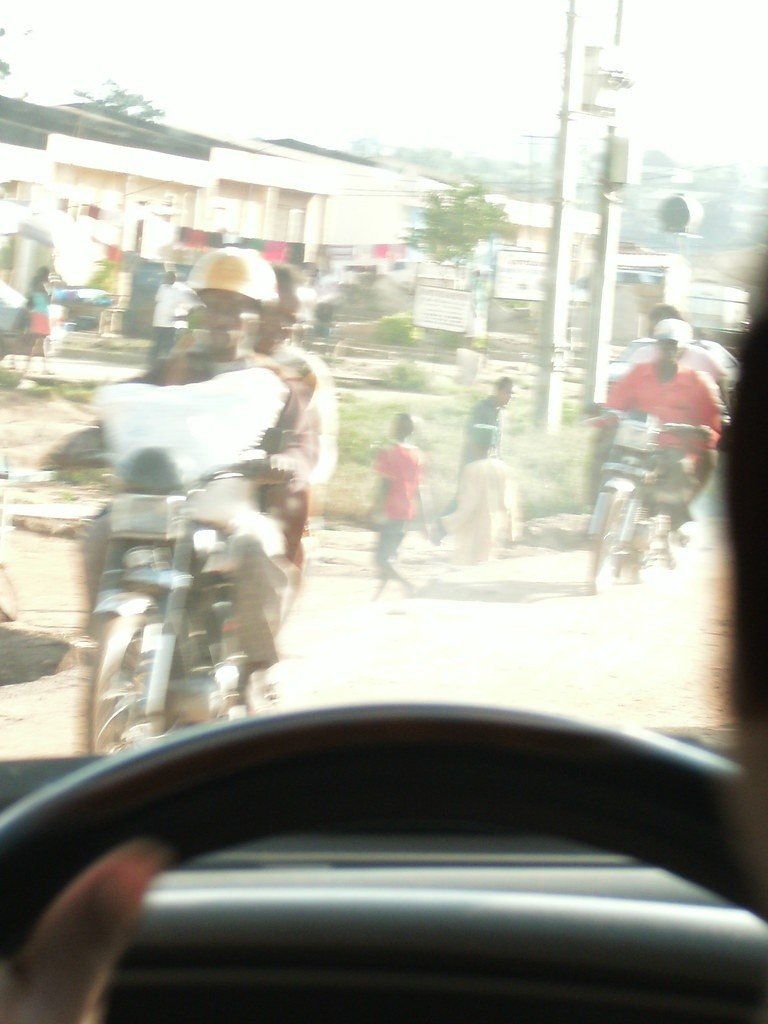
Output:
[0,243,524,719]
[580,301,739,554]
[0,312,768,1023]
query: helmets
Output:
[183,247,279,304]
[655,318,694,352]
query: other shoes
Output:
[237,670,275,719]
[656,540,676,570]
[673,529,689,547]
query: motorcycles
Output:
[43,453,281,760]
[584,406,709,600]
